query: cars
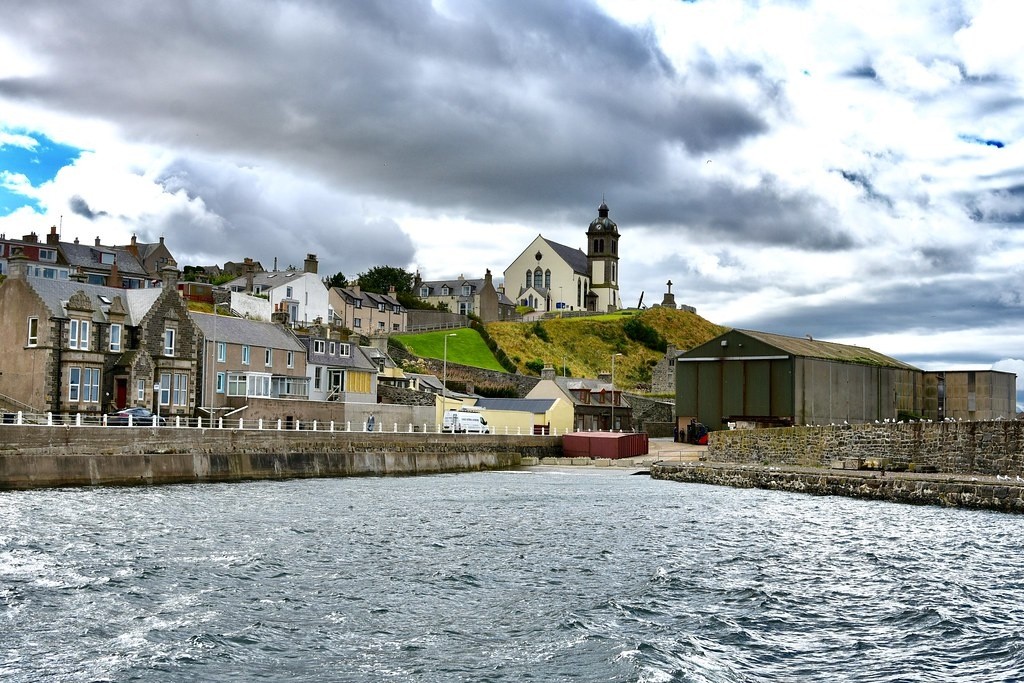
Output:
[101,406,167,427]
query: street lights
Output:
[440,333,458,434]
[611,353,622,430]
[209,302,229,428]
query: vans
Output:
[443,407,489,434]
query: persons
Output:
[680,428,686,443]
[367,412,375,431]
